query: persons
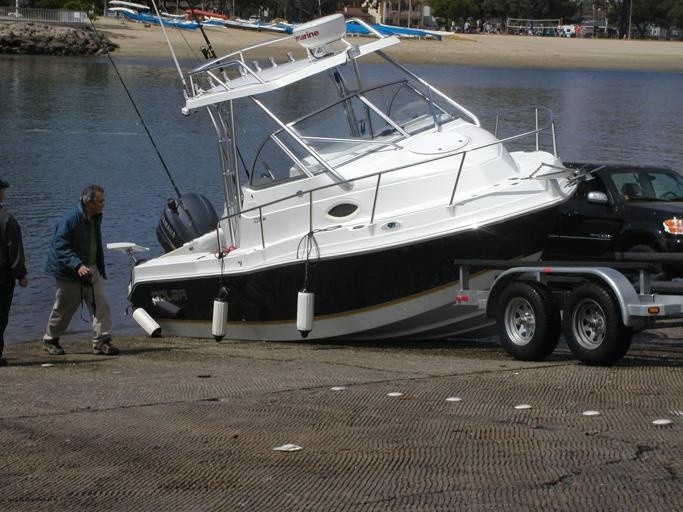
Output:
[42,184,120,355]
[0,179,28,365]
[444,15,583,38]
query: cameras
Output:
[79,272,93,288]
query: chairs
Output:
[621,183,640,199]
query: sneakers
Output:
[93,338,119,355]
[43,338,64,355]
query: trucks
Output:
[455,257,682,367]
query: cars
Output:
[531,161,683,280]
